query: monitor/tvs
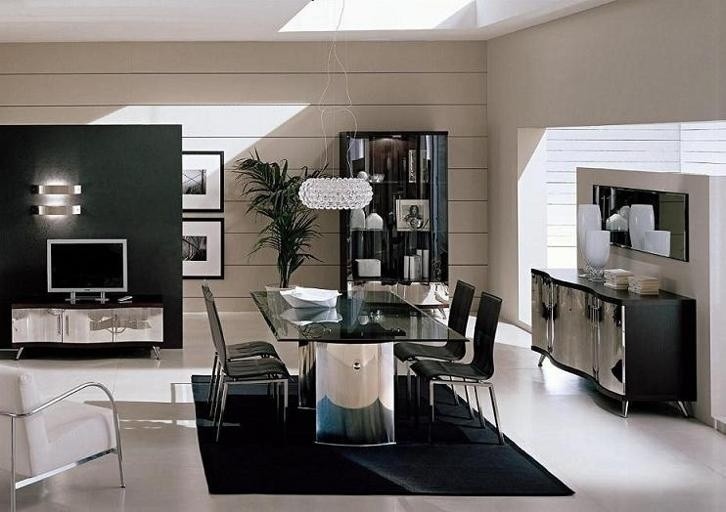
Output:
[46,239,128,301]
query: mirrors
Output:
[592,184,690,263]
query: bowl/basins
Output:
[279,309,344,327]
[368,173,385,183]
[278,286,343,309]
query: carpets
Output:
[191,374,575,496]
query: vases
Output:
[577,202,601,278]
[586,229,611,284]
[629,205,671,257]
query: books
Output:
[604,269,659,295]
[404,249,429,279]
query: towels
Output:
[603,269,660,295]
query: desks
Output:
[248,289,473,447]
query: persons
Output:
[405,205,423,222]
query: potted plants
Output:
[225,145,334,319]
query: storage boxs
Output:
[354,258,382,277]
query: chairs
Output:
[201,285,282,416]
[408,291,503,445]
[393,281,476,409]
[0,366,126,512]
[205,294,297,443]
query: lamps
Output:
[33,184,82,217]
[298,0,384,232]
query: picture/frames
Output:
[181,218,225,279]
[181,151,225,213]
[395,199,430,233]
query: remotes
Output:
[117,300,132,304]
[117,295,133,301]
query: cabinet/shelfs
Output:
[531,267,697,418]
[337,131,448,319]
[12,304,165,361]
